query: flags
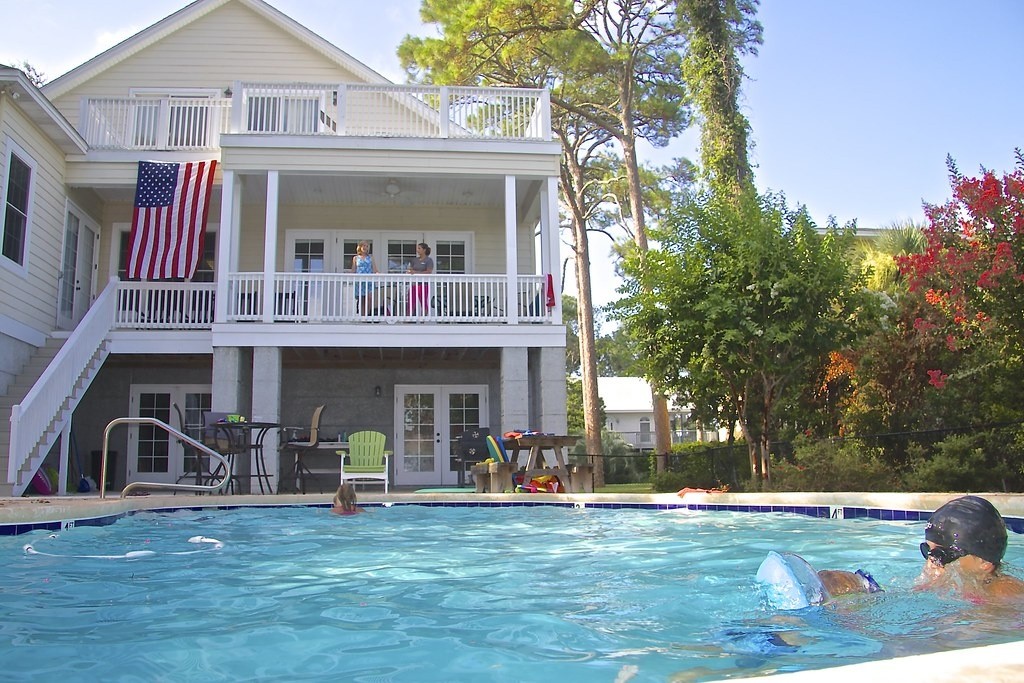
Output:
[125,160,217,279]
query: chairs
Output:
[378,284,531,323]
[459,426,489,488]
[173,402,223,495]
[335,429,394,495]
[277,404,328,493]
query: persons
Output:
[330,484,366,515]
[672,570,865,683]
[349,240,379,316]
[408,243,434,316]
[913,495,1024,642]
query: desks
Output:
[503,435,582,492]
[207,421,280,494]
[289,442,367,491]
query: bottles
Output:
[342,431,346,442]
[337,433,341,442]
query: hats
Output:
[926,495,1009,565]
[757,550,829,610]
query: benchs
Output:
[564,464,593,491]
[469,461,518,493]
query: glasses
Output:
[919,542,969,567]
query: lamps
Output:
[375,385,380,395]
[385,181,400,195]
[7,86,20,99]
